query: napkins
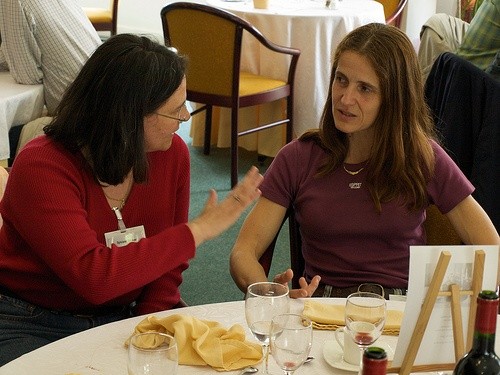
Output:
[123,314,266,371]
[301,300,401,336]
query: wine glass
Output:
[344,292,387,375]
[269,314,313,375]
[245,282,290,375]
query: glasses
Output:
[150,100,188,124]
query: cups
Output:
[335,322,376,364]
[127,332,178,375]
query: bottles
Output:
[359,347,388,375]
[452,290,500,375]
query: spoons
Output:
[239,366,258,375]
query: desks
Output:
[0,299,500,375]
[190,0,388,166]
[0,69,44,168]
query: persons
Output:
[229,23,500,300]
[416,0,500,87]
[0,0,103,161]
[0,34,264,369]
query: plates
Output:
[322,331,394,371]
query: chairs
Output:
[257,201,467,289]
[77,0,119,38]
[377,0,409,29]
[160,1,302,189]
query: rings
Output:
[233,194,238,199]
